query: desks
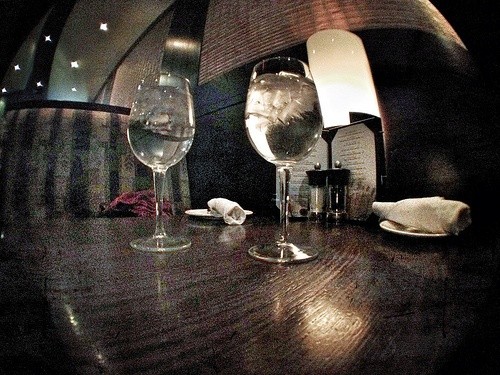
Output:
[0,217,500,375]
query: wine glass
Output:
[126,72,195,252]
[245,57,322,261]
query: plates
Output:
[185,208,253,217]
[380,220,450,236]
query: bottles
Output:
[324,161,350,224]
[306,163,325,223]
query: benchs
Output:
[0,100,192,222]
